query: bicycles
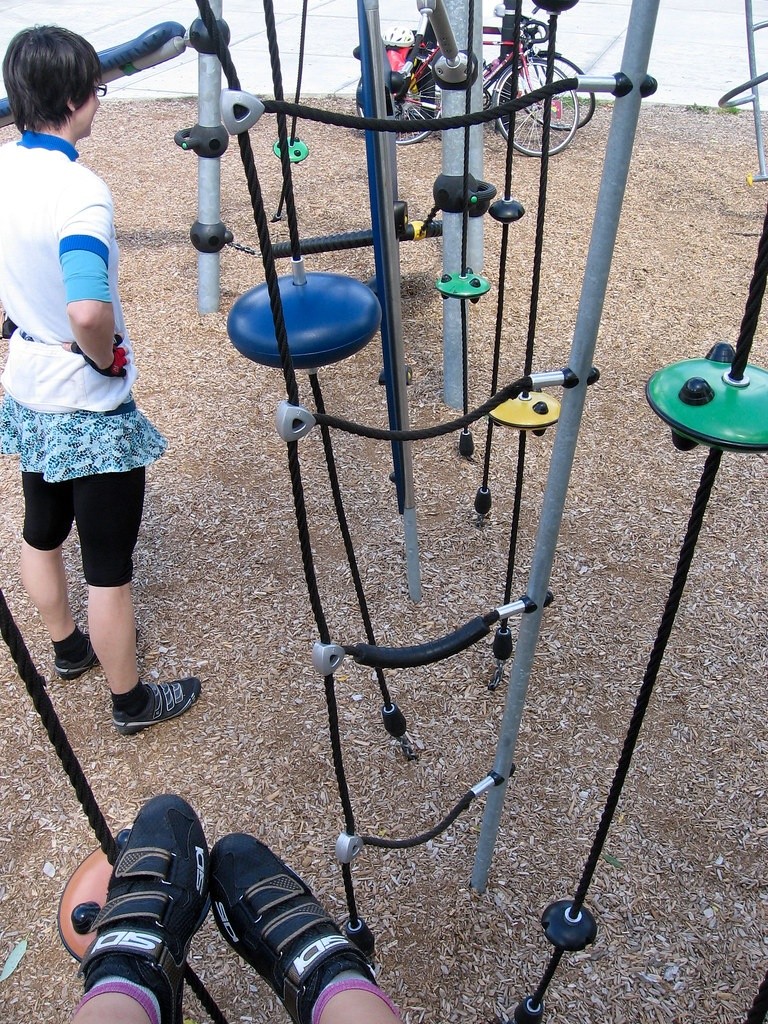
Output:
[356,0,595,160]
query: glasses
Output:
[92,83,107,99]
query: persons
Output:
[67,795,407,1024]
[0,26,201,735]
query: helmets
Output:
[383,26,414,47]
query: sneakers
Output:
[113,677,200,735]
[211,833,377,1024]
[53,628,138,680]
[77,794,210,1024]
